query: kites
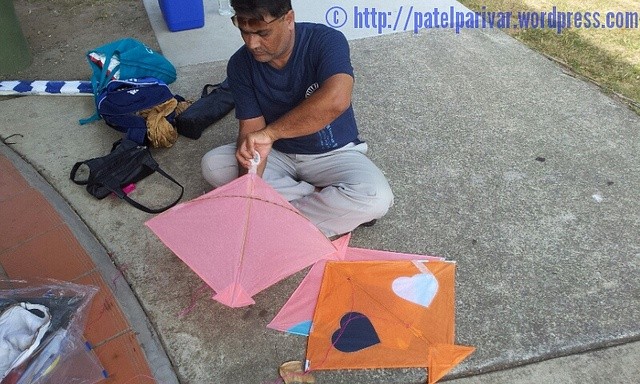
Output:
[144,151,339,307]
[304,260,474,382]
[266,246,445,337]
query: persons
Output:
[200,1,395,238]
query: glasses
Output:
[230,13,287,31]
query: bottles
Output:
[219,1,232,17]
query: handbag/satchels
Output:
[97,77,186,145]
[176,77,235,139]
[86,38,177,86]
[70,138,184,214]
